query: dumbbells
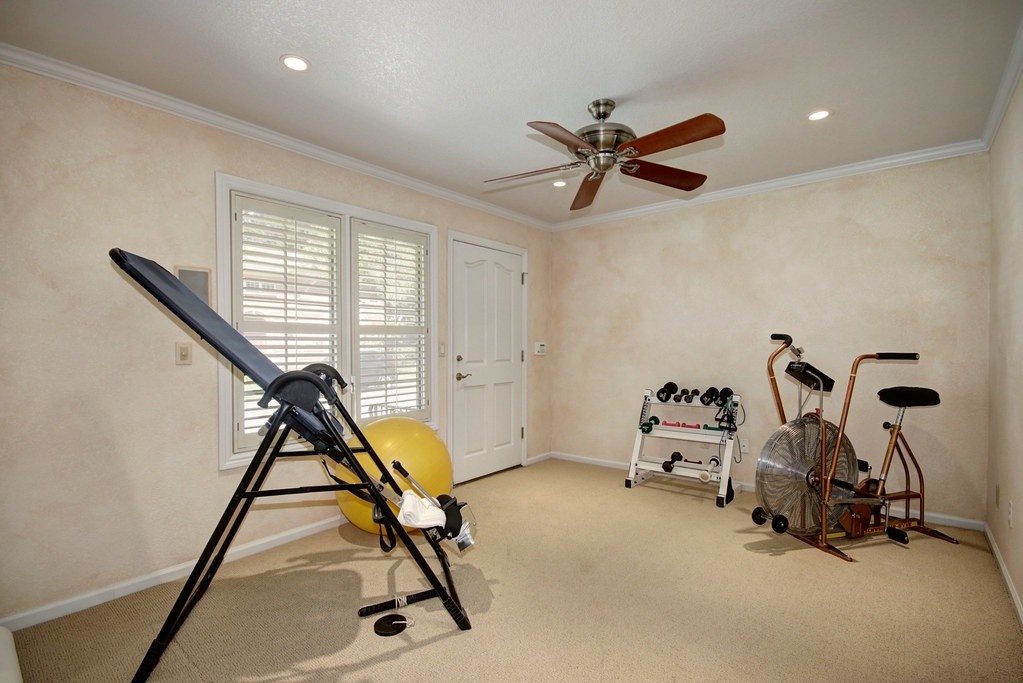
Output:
[662,420,680,427]
[714,388,733,407]
[673,388,689,402]
[683,388,700,403]
[641,416,660,434]
[656,382,678,402]
[684,458,702,464]
[703,423,723,431]
[662,451,683,472]
[682,422,700,429]
[700,387,719,406]
[700,455,720,483]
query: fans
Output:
[484,97,727,211]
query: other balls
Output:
[335,417,453,536]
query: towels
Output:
[395,488,448,531]
